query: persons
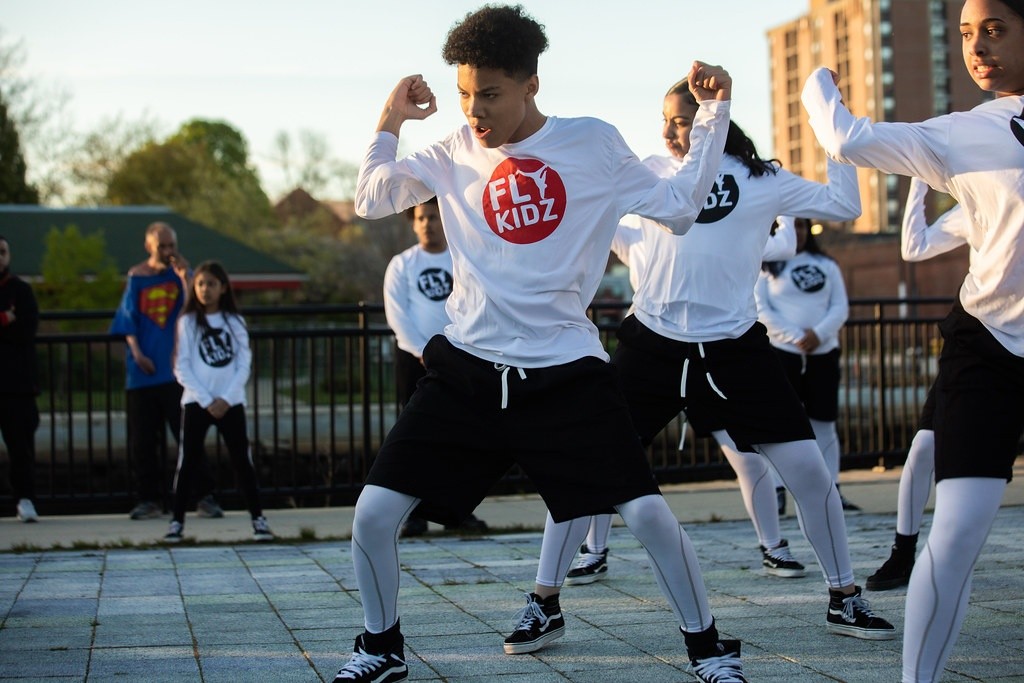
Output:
[0,235,39,523]
[108,221,225,521]
[332,4,744,683]
[801,0,1024,683]
[163,262,274,542]
[383,196,488,537]
[502,77,895,654]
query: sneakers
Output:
[776,485,788,519]
[504,591,565,654]
[162,519,185,542]
[17,500,38,522]
[866,530,920,591]
[198,494,224,519]
[679,617,746,683]
[564,545,609,585]
[250,514,275,540]
[834,484,865,515]
[131,503,163,520]
[760,538,804,578]
[826,586,896,639]
[332,617,408,683]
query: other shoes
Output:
[398,518,429,537]
[444,514,489,531]
